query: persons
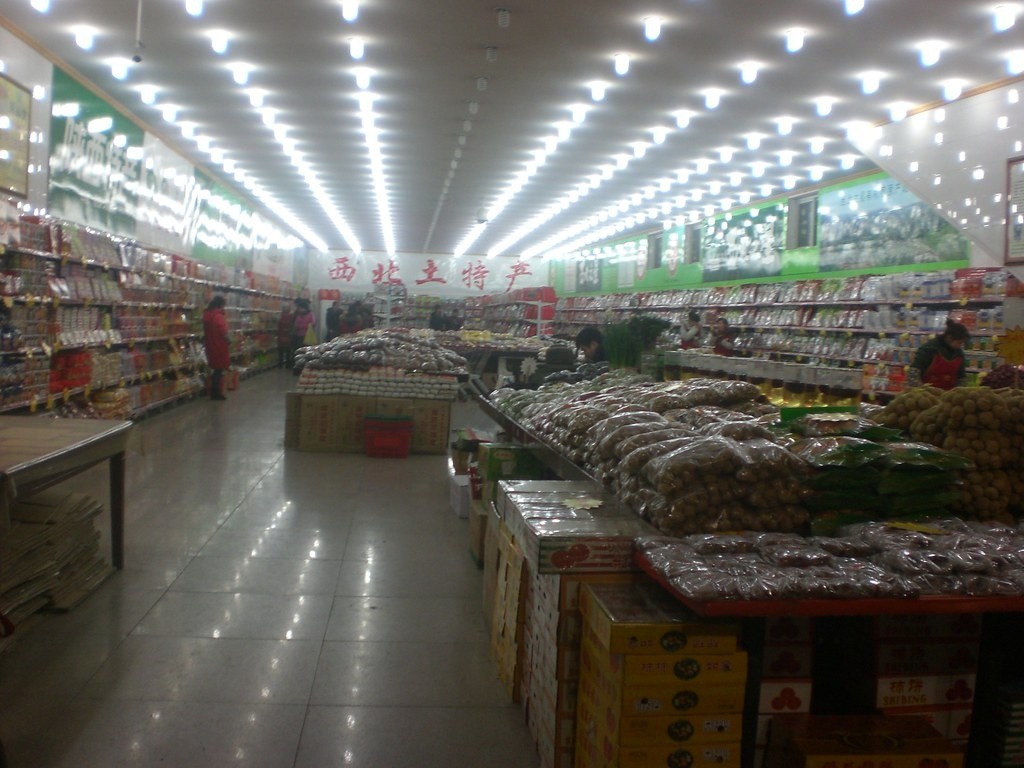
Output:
[277,298,315,368]
[204,296,231,400]
[709,318,734,356]
[327,301,373,339]
[680,314,703,350]
[577,328,606,361]
[907,318,969,390]
[430,305,463,330]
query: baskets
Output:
[362,413,412,459]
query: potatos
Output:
[872,384,1024,522]
[550,380,815,536]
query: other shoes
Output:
[211,393,226,400]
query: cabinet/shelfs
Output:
[340,272,1023,391]
[1,247,301,422]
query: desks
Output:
[1,414,136,638]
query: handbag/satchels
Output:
[303,322,318,346]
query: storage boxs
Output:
[282,393,454,457]
[445,424,1024,768]
